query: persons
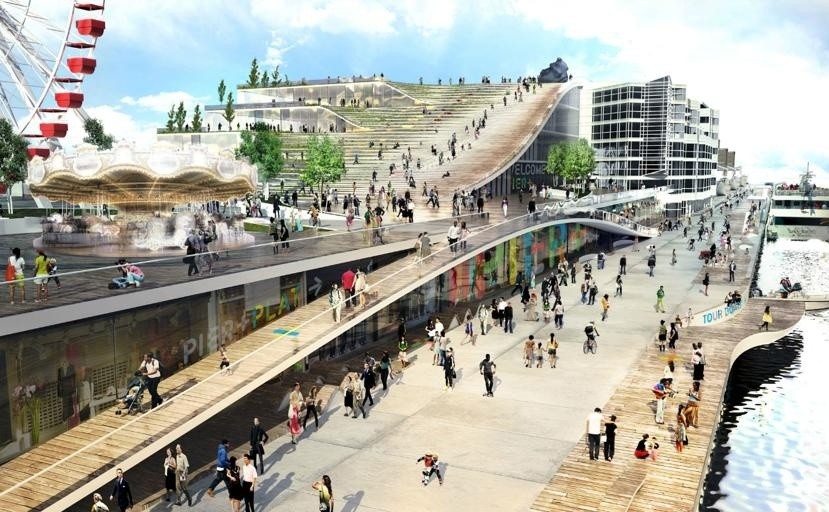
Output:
[550,280,706,462]
[544,178,761,296]
[425,296,559,396]
[724,291,773,331]
[116,352,165,409]
[91,417,270,512]
[1,73,544,305]
[218,344,229,374]
[416,447,443,486]
[312,474,334,511]
[779,276,792,293]
[75,366,94,422]
[329,266,368,323]
[779,183,793,195]
[287,315,409,445]
